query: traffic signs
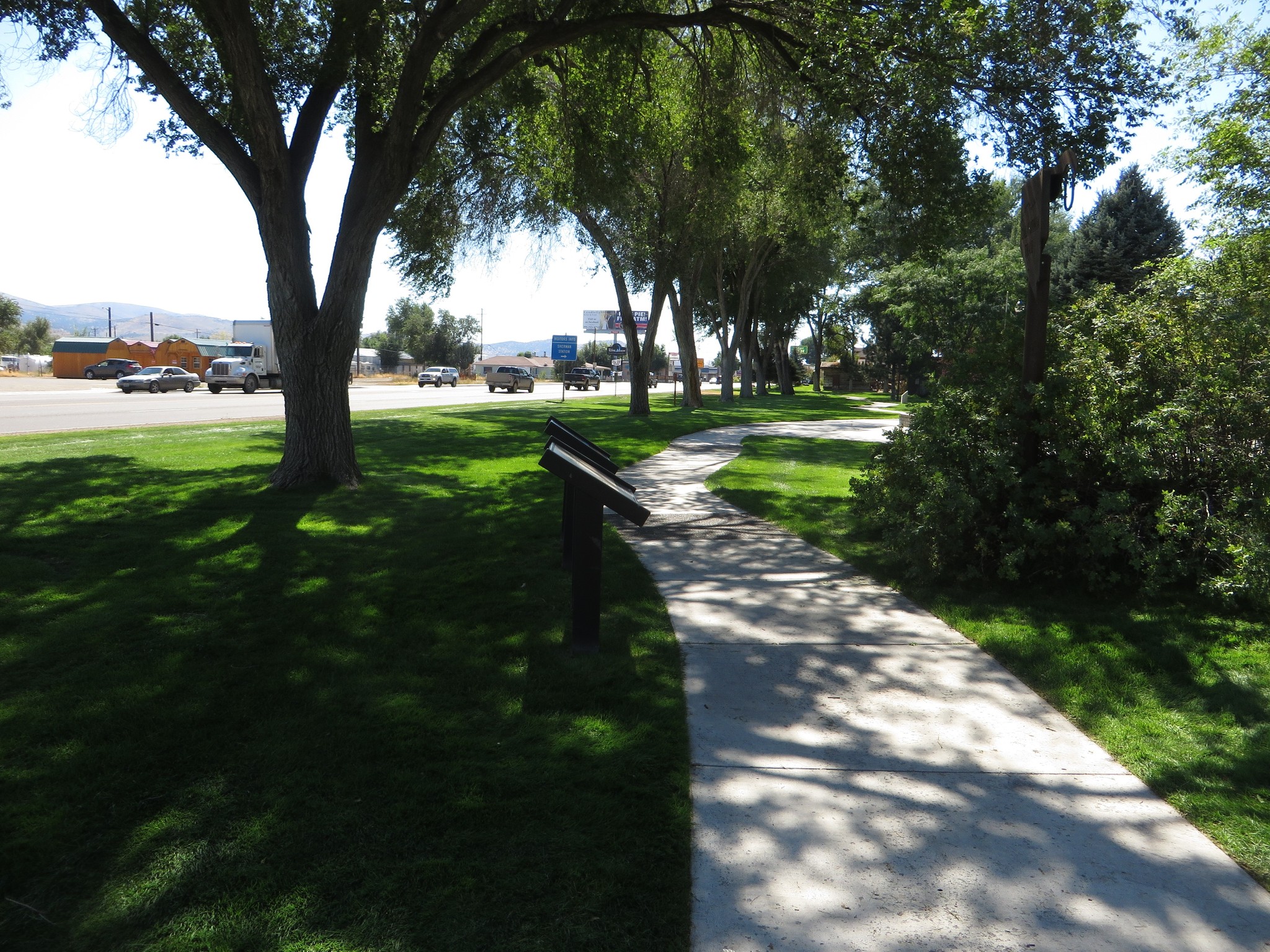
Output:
[551,335,578,361]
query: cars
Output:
[116,366,201,393]
[716,375,722,384]
[709,377,717,384]
[0,354,20,372]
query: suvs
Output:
[417,366,460,388]
[83,358,143,380]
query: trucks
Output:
[564,367,601,391]
[648,372,658,388]
[204,320,353,394]
[485,366,535,393]
[676,374,683,382]
